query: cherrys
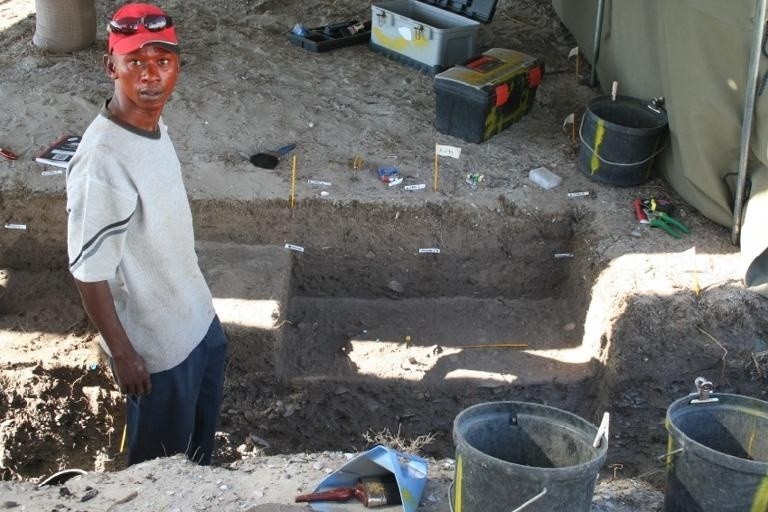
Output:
[250,144,295,168]
[296,476,400,506]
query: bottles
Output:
[107,2,178,57]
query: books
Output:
[426,48,547,145]
[364,0,500,79]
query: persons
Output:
[65,2,228,469]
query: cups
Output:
[107,15,173,35]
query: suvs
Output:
[574,95,668,188]
[662,376,767,512]
[451,400,609,512]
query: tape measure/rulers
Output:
[639,204,691,239]
